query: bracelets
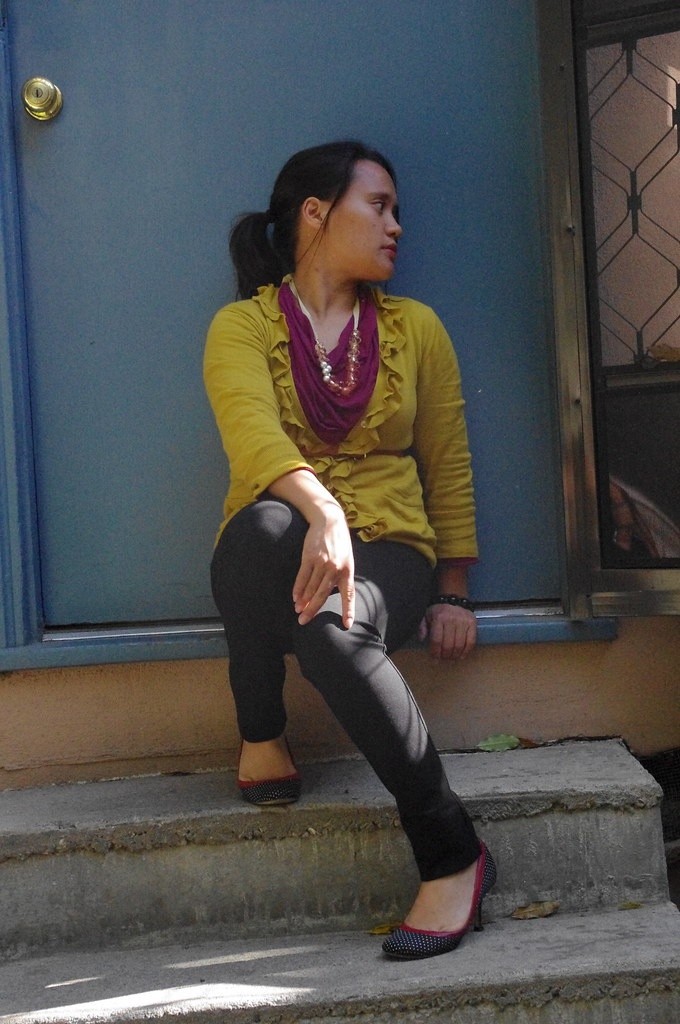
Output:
[428,593,472,612]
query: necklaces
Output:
[284,272,362,396]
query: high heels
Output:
[381,839,497,960]
[237,737,302,807]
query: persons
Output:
[202,140,494,959]
